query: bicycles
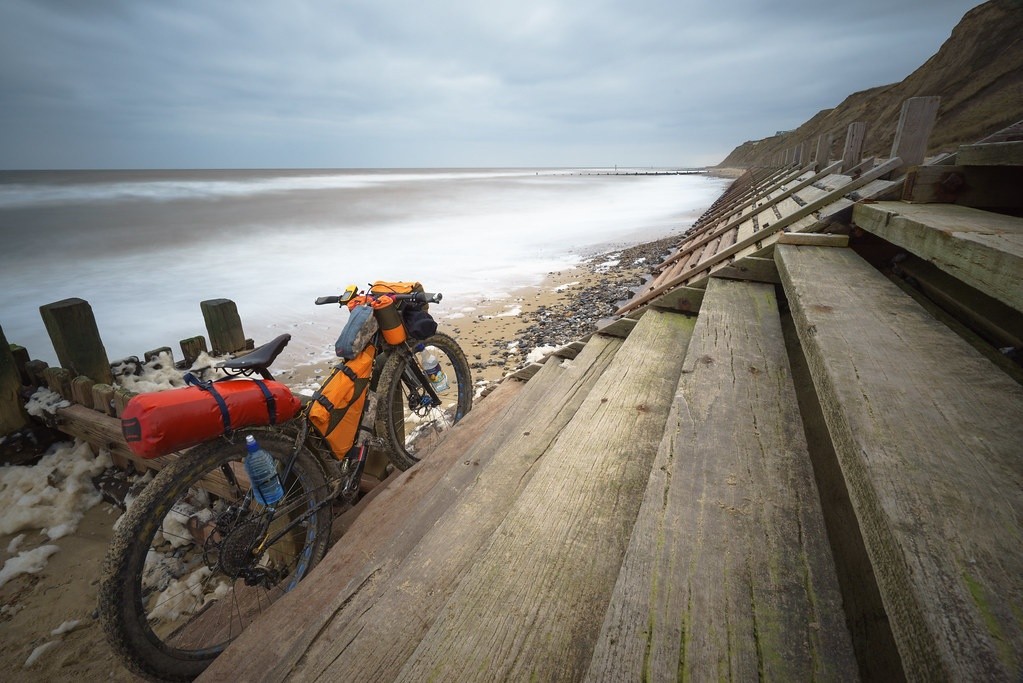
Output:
[97,285,474,683]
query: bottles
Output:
[348,297,367,316]
[244,435,284,505]
[417,343,447,385]
[372,295,407,345]
